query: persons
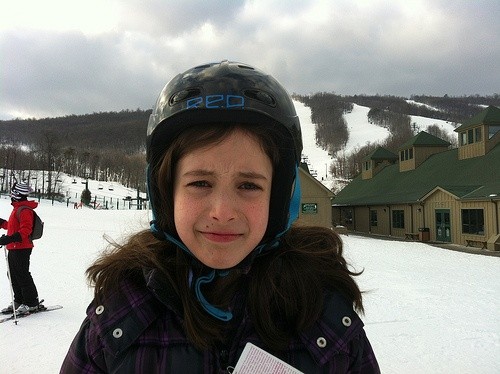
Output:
[59,61,380,373]
[0,181,40,315]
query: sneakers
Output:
[7,301,21,311]
[17,304,38,313]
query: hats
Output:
[11,181,30,200]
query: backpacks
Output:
[17,206,44,239]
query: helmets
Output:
[146,59,303,179]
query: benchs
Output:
[405,233,419,240]
[465,239,488,249]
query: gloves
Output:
[0,236,12,245]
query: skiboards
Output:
[0,298,64,323]
